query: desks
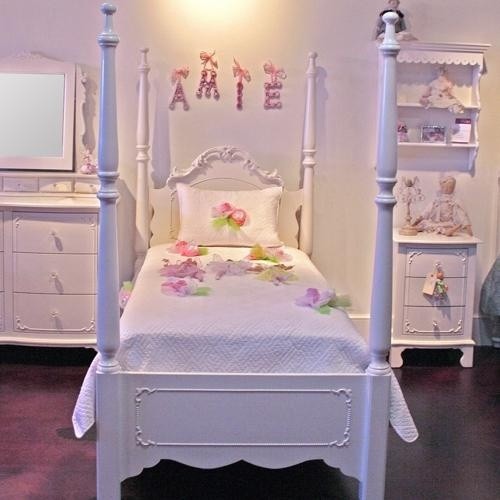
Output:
[0,172,123,353]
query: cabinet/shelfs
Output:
[389,228,484,370]
[378,36,491,172]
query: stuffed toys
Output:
[370,0,407,40]
[398,121,410,143]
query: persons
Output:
[431,271,448,300]
[426,131,438,143]
[432,128,445,140]
[410,174,475,238]
[419,64,466,115]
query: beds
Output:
[94,0,400,499]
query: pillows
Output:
[176,181,284,249]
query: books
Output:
[450,117,472,144]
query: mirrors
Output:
[0,49,74,172]
